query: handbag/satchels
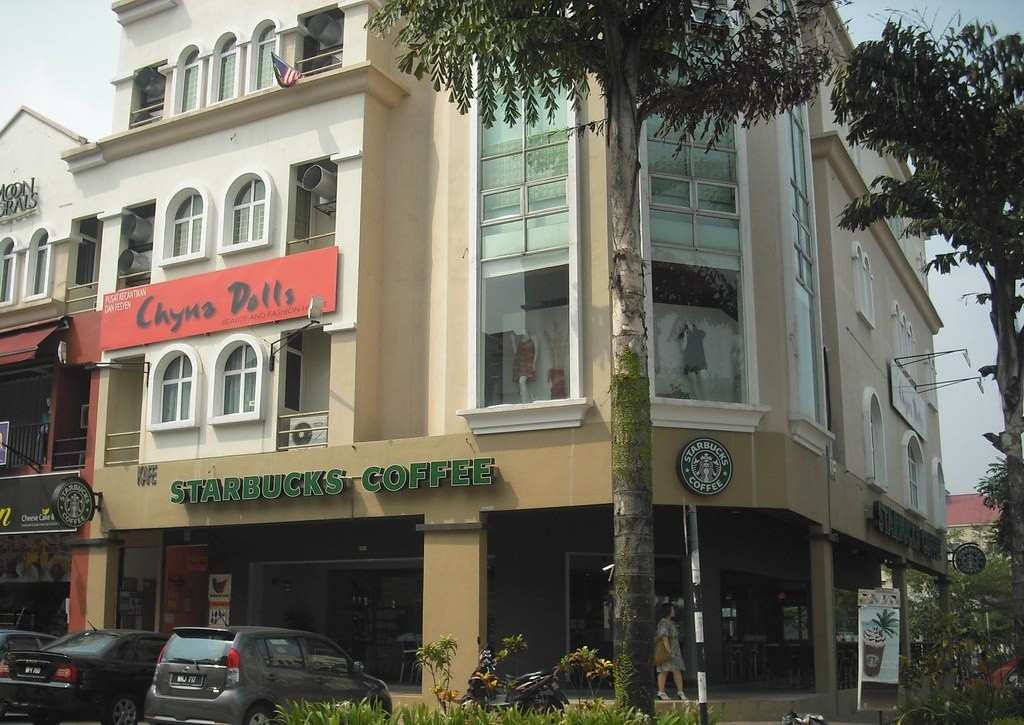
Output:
[654,638,671,664]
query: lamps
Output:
[267,298,321,372]
[895,348,972,368]
[58,341,151,388]
[914,375,985,395]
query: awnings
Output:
[0,314,74,366]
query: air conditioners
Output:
[288,415,328,451]
[80,405,89,429]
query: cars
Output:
[0,628,173,725]
[0,629,60,719]
[960,654,1024,702]
[144,625,394,725]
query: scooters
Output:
[459,636,571,725]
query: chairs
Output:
[398,632,422,685]
[415,633,422,684]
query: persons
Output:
[510,329,539,404]
[36,396,52,465]
[680,319,711,401]
[653,602,688,701]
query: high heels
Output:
[657,691,671,700]
[676,691,688,700]
